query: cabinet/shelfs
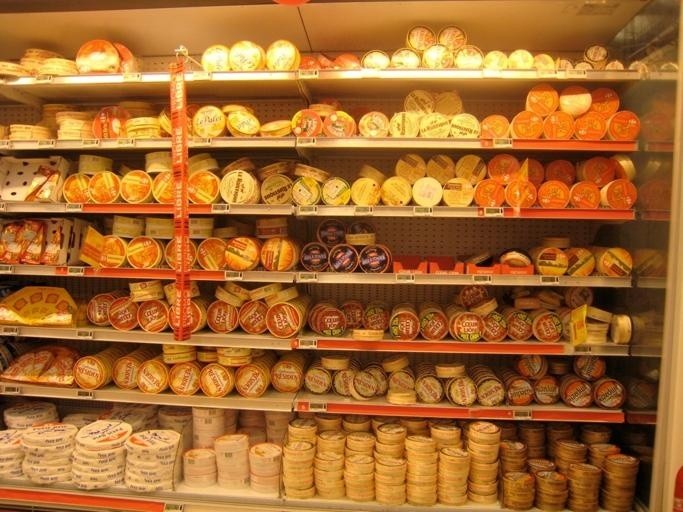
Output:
[0,71,683,512]
